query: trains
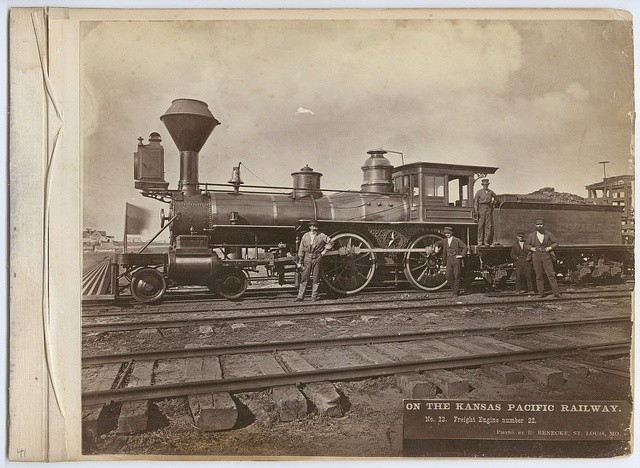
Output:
[117,99,634,303]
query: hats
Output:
[518,231,525,236]
[535,217,544,224]
[481,179,490,184]
[443,227,453,233]
[308,219,320,226]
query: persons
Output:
[511,228,536,296]
[526,218,564,300]
[433,227,467,298]
[474,180,499,246]
[291,221,334,303]
